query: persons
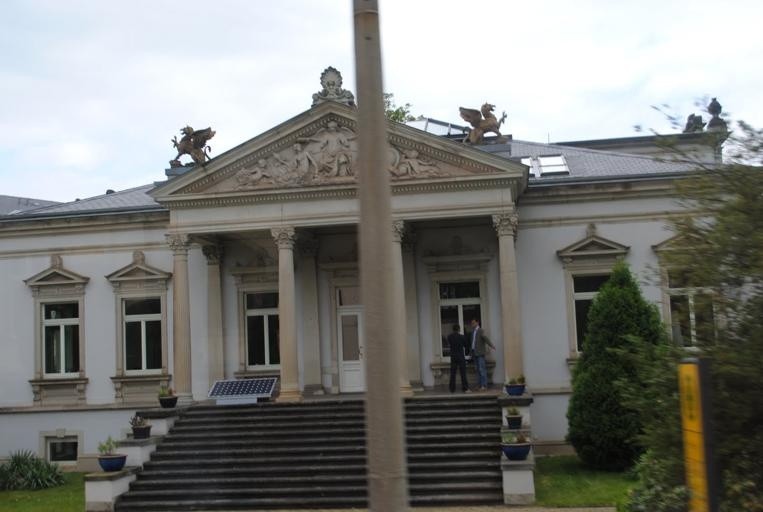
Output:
[234,121,439,188]
[469,319,496,392]
[448,324,472,394]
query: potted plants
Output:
[505,373,526,395]
[157,384,179,408]
[499,435,531,460]
[504,406,523,428]
[128,414,152,439]
[95,435,128,472]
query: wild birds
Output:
[707,98,722,118]
